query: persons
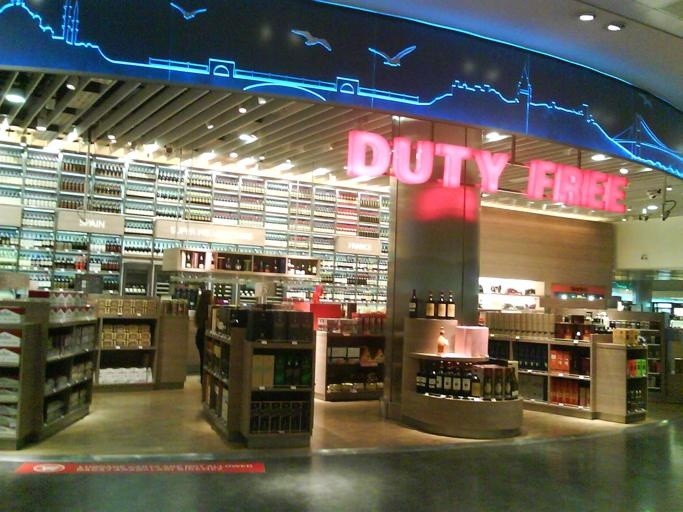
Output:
[194,282,214,384]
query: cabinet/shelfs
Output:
[0,142,683,451]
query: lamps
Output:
[206,97,267,129]
[6,77,79,132]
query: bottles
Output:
[0,148,388,304]
[407,288,457,319]
[437,326,448,356]
[416,360,519,401]
